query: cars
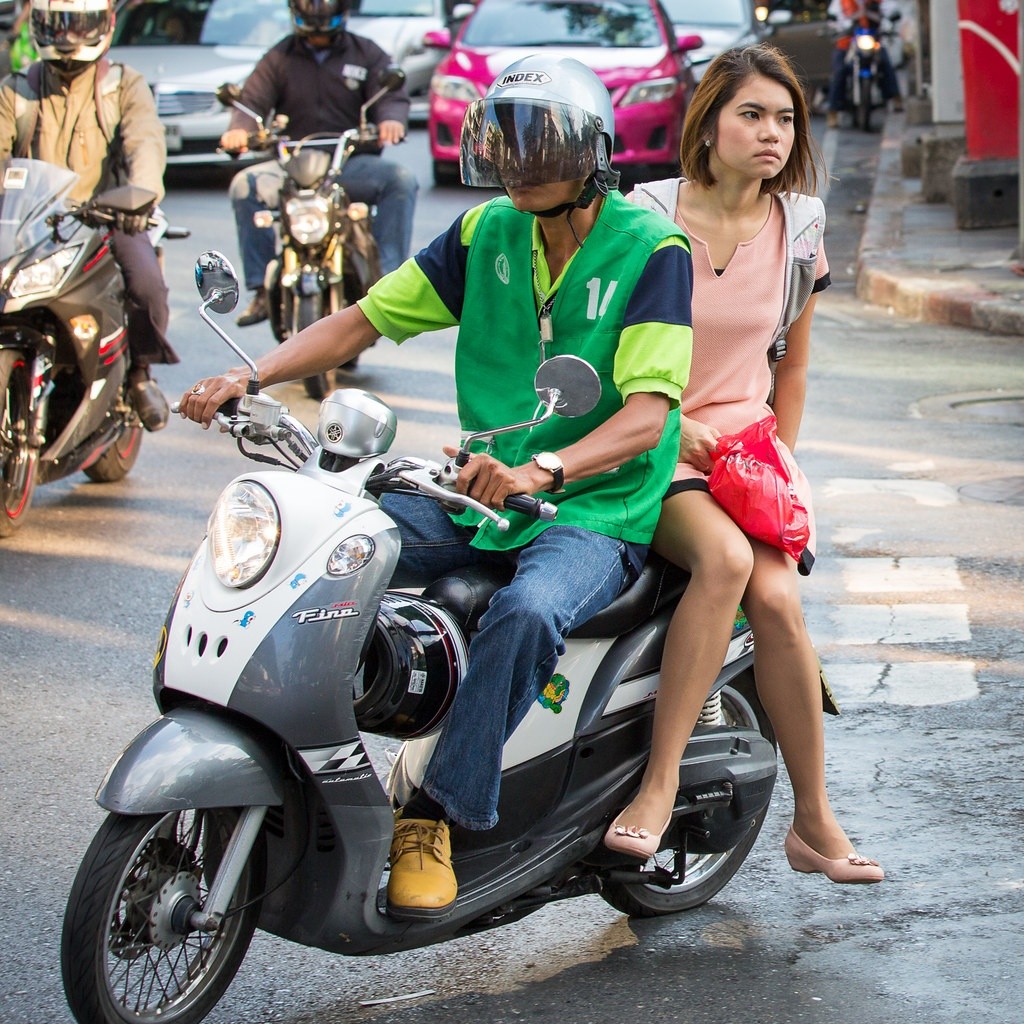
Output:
[344,0,475,121]
[664,0,793,89]
[100,0,298,166]
[422,0,703,185]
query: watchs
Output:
[531,451,566,494]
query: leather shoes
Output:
[385,804,458,918]
[129,367,169,431]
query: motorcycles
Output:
[1,158,190,536]
[815,11,900,132]
[216,64,407,399]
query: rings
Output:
[704,466,709,471]
[191,383,205,394]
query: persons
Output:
[221,0,421,327]
[176,50,693,918]
[600,47,885,881]
[0,0,180,430]
[826,0,904,129]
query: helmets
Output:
[476,52,615,165]
[27,0,115,73]
[346,590,469,742]
[287,0,350,38]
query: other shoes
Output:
[605,802,675,860]
[237,289,269,328]
[892,98,902,112]
[784,825,884,885]
[826,111,837,126]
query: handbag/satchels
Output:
[709,412,808,564]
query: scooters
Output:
[60,251,842,1024]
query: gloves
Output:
[105,203,154,236]
